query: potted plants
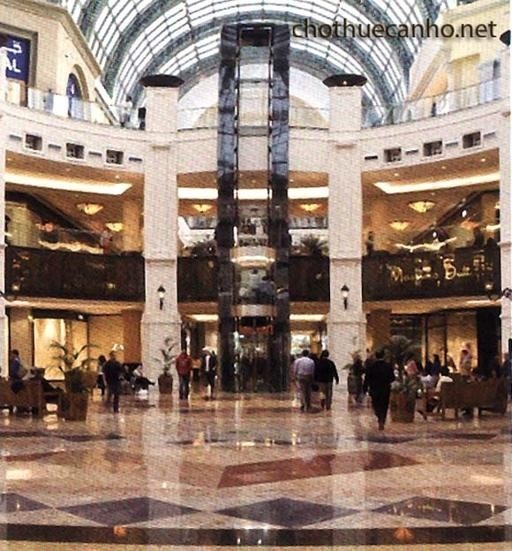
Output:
[153,343,178,393]
[375,335,421,423]
[44,340,101,420]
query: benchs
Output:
[418,382,508,421]
[0,377,44,415]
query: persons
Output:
[365,231,374,253]
[9,350,511,431]
[122,97,133,123]
[37,222,60,251]
